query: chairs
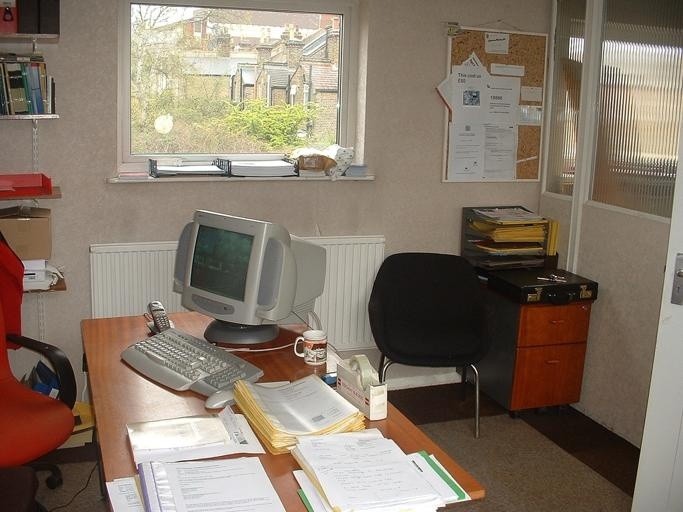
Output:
[368,252,486,439]
[0,232,77,512]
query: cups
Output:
[294,330,327,365]
[543,251,559,271]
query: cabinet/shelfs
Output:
[456,267,598,419]
[0,32,66,292]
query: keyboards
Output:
[120,327,264,397]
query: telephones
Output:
[145,300,173,334]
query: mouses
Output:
[205,390,237,409]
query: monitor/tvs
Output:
[172,209,327,348]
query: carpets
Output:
[36,415,633,512]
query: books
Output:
[467,207,558,271]
[231,374,367,456]
[0,51,57,116]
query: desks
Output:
[79,311,484,512]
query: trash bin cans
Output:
[50,424,95,461]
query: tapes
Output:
[348,355,373,388]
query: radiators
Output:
[89,236,385,351]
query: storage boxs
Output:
[22,260,48,281]
[0,206,52,260]
